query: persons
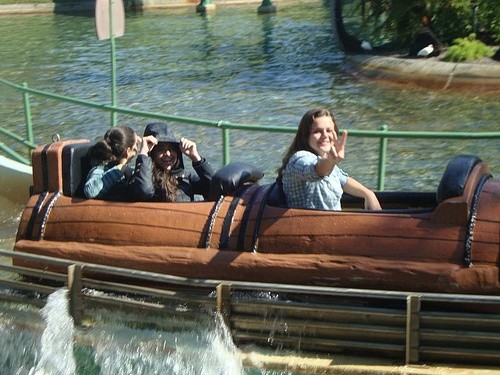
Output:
[276,108,382,212]
[82,125,143,202]
[128,120,223,202]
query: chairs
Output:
[63,140,98,197]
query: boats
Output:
[10,132,500,347]
